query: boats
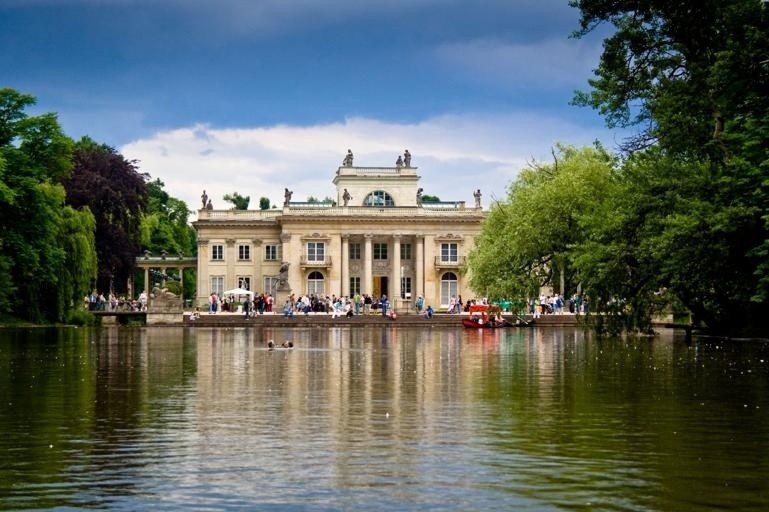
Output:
[460,304,506,328]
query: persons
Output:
[417,189,424,208]
[404,150,411,167]
[396,156,403,167]
[201,190,207,209]
[342,188,352,207]
[281,341,293,348]
[84,288,149,313]
[285,189,293,206]
[267,341,276,352]
[343,149,353,167]
[475,190,481,209]
[207,200,213,210]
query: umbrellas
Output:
[224,288,254,303]
